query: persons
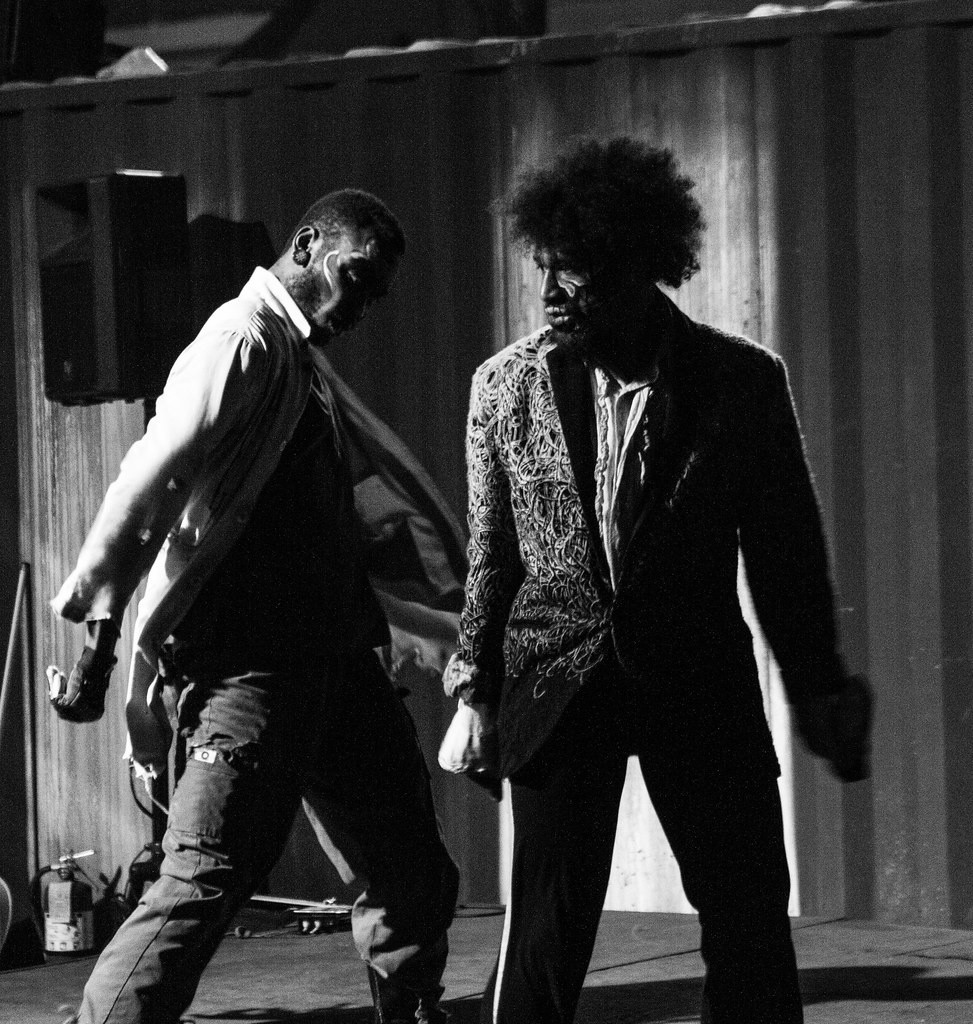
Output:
[438,140,876,1024]
[45,190,460,1024]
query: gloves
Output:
[46,645,119,723]
[438,699,502,802]
[795,671,875,784]
